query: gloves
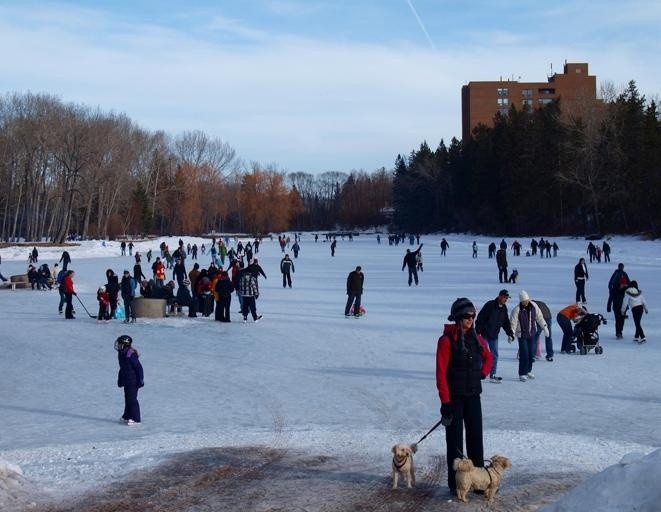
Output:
[507,337,513,344]
[508,334,514,341]
[544,326,549,337]
[440,402,453,420]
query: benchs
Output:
[11,274,54,290]
[165,298,190,318]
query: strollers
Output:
[566,312,608,355]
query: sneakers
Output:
[244,316,247,320]
[547,358,553,361]
[131,318,136,323]
[489,374,503,381]
[125,318,129,322]
[633,337,639,342]
[120,417,126,420]
[520,375,528,382]
[527,372,534,379]
[128,419,136,422]
[255,316,262,322]
[638,338,647,342]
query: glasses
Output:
[462,312,475,319]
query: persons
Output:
[60,250,70,272]
[508,291,550,381]
[415,252,424,272]
[601,241,611,263]
[608,261,631,289]
[440,237,449,255]
[525,238,560,257]
[620,280,649,344]
[344,264,364,317]
[607,276,630,339]
[508,267,518,283]
[330,240,337,257]
[574,257,589,303]
[27,264,62,290]
[488,242,497,259]
[594,244,602,263]
[531,299,556,362]
[313,231,358,243]
[471,240,479,259]
[106,270,120,319]
[436,297,494,496]
[497,246,510,282]
[63,271,78,319]
[97,286,109,318]
[120,240,134,257]
[500,238,508,248]
[32,247,39,263]
[279,254,295,290]
[556,304,586,353]
[28,253,32,263]
[121,271,137,322]
[586,241,596,263]
[474,289,514,383]
[376,231,421,246]
[401,242,423,286]
[511,240,523,256]
[277,230,303,257]
[134,236,266,322]
[114,336,146,426]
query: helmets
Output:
[115,335,132,352]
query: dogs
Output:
[452,454,512,503]
[391,443,418,490]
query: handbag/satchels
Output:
[113,302,124,318]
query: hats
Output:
[448,298,476,321]
[499,290,511,298]
[181,280,190,286]
[99,286,106,293]
[519,291,529,302]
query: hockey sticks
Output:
[75,294,97,319]
